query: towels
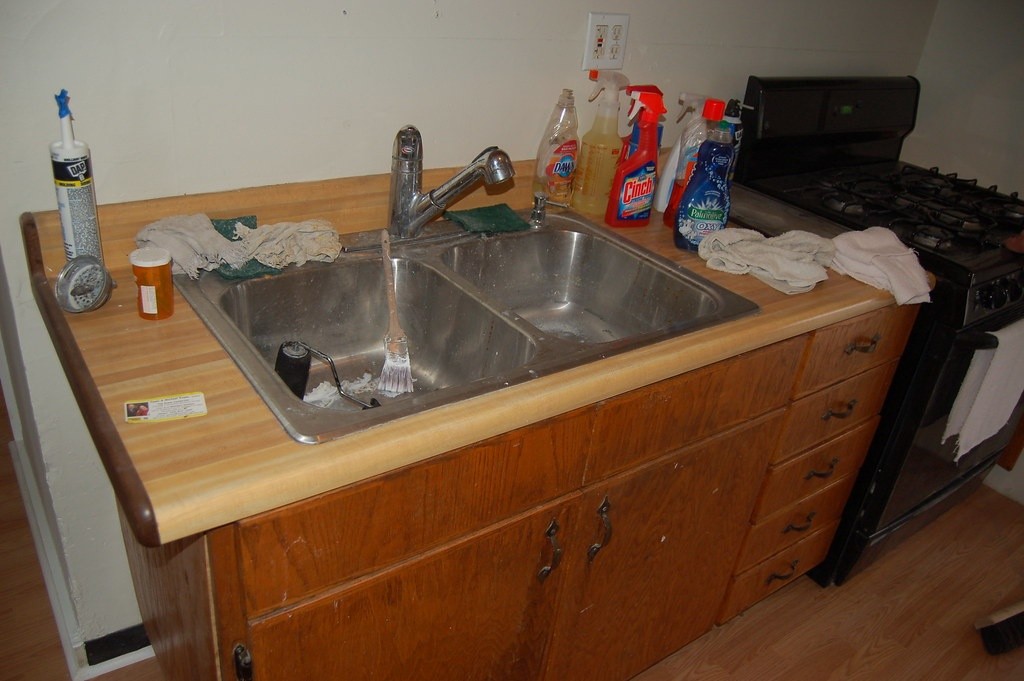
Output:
[940,316,1024,469]
[824,226,934,308]
[699,225,839,299]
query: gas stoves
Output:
[726,75,1024,328]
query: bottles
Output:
[130,247,175,320]
[49,93,104,273]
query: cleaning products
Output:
[628,119,663,161]
[531,88,581,207]
[670,118,733,252]
[604,91,666,235]
[610,80,663,186]
[652,88,711,214]
[662,98,726,232]
[580,63,626,218]
[710,100,754,196]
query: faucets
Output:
[390,123,519,249]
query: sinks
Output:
[410,200,766,370]
[169,239,568,447]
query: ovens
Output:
[804,311,1024,591]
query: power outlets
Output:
[586,7,632,74]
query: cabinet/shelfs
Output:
[117,290,932,681]
